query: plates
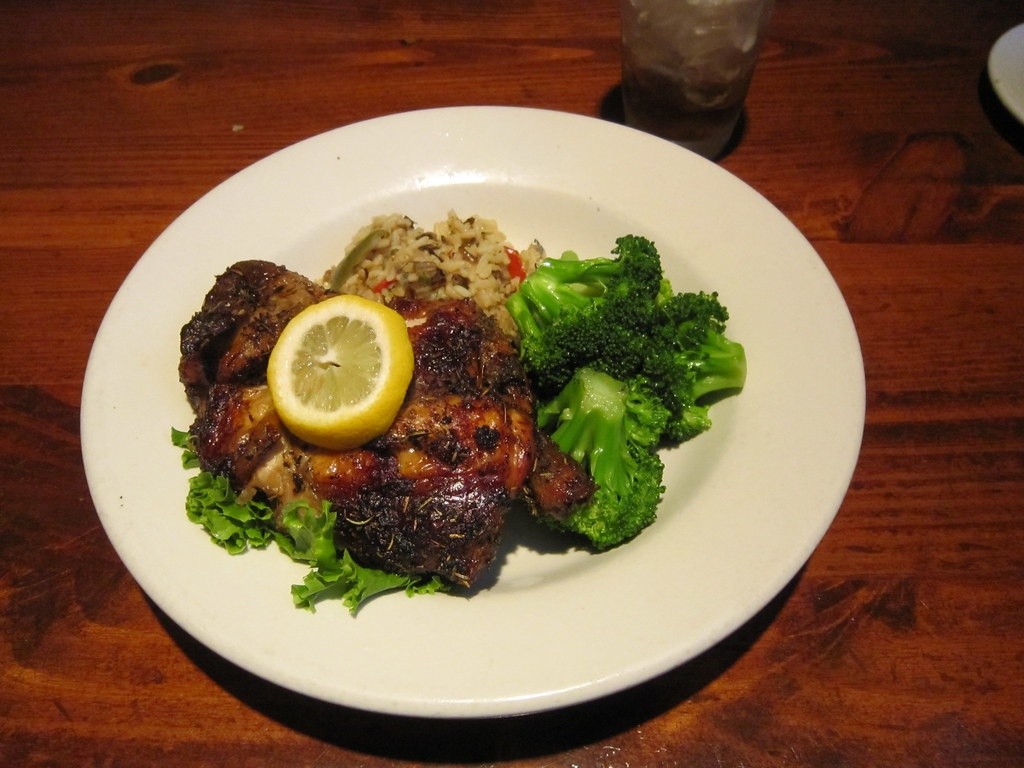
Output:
[987,23,1024,124]
[80,107,868,716]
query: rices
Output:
[320,208,545,326]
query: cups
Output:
[618,0,772,162]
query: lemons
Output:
[266,295,414,452]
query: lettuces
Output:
[170,426,456,615]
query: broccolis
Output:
[504,232,749,549]
